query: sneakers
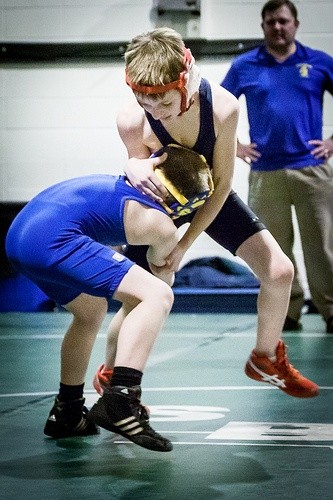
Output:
[86,386,173,452]
[244,340,318,399]
[43,395,100,437]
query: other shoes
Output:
[326,318,333,333]
[283,317,304,332]
